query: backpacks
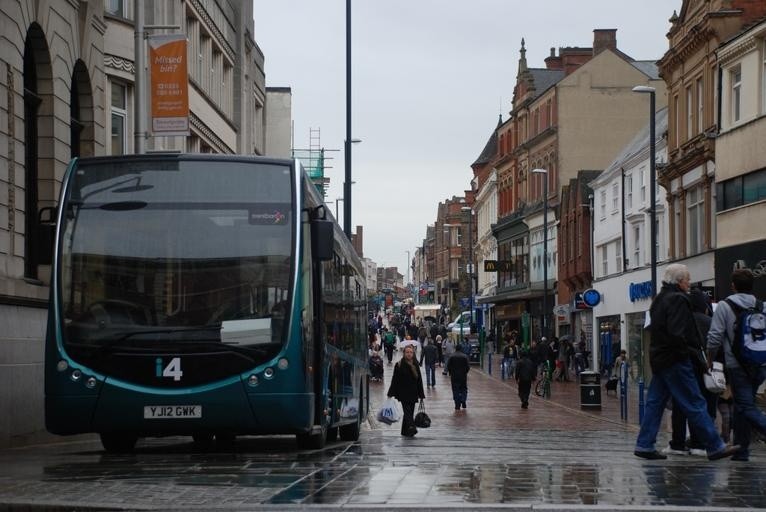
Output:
[723,296,766,362]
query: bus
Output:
[33,149,369,456]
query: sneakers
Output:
[663,446,686,457]
[730,453,750,462]
[707,443,741,462]
[521,400,529,408]
[455,401,467,410]
[690,448,706,457]
[634,448,669,461]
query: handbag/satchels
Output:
[704,359,727,393]
[413,399,431,429]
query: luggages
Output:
[605,378,619,395]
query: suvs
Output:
[447,311,476,331]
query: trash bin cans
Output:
[579,372,602,412]
[467,335,481,366]
[369,311,373,319]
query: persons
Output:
[705,268,765,461]
[661,284,716,456]
[633,263,747,460]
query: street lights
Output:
[530,166,550,338]
[415,245,421,302]
[460,205,475,326]
[405,249,409,286]
[333,135,363,226]
[632,81,661,301]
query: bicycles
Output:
[535,360,552,398]
